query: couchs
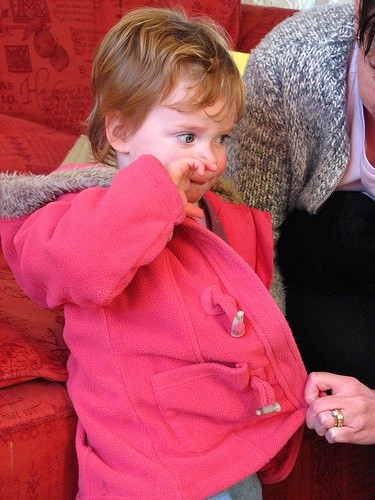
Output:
[0,0,374,500]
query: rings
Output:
[330,408,344,426]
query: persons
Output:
[214,0,375,447]
[0,7,331,500]
